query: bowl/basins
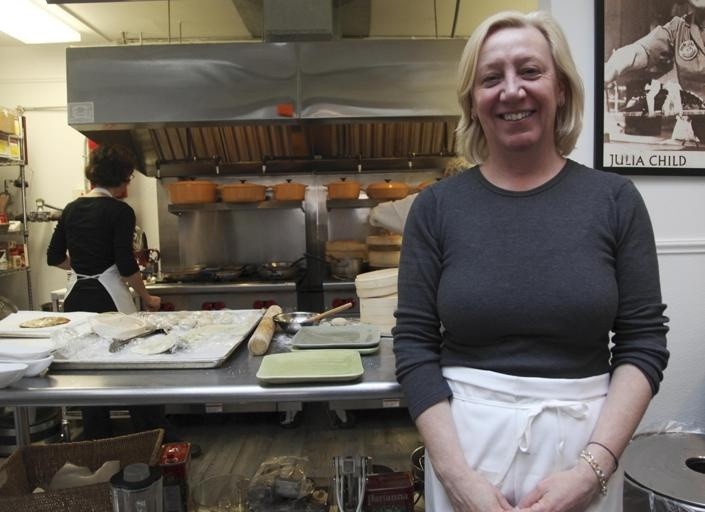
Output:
[0,363,27,390]
[0,339,53,377]
[272,312,321,335]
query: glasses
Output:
[127,174,135,181]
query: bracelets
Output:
[578,449,608,499]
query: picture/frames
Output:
[594,0,705,177]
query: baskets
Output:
[1,427,164,510]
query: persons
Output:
[45,140,202,458]
[606,0,705,145]
[389,10,671,512]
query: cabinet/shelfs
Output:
[0,107,35,311]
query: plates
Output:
[0,311,97,338]
[50,309,264,369]
[291,325,380,354]
[256,349,364,384]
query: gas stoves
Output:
[141,269,310,314]
[321,274,358,319]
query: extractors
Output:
[65,1,470,177]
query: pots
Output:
[416,178,442,191]
[215,180,271,203]
[330,258,361,280]
[256,262,295,280]
[366,179,409,199]
[323,177,363,200]
[271,179,308,201]
[162,178,217,205]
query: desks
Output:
[0,308,405,402]
